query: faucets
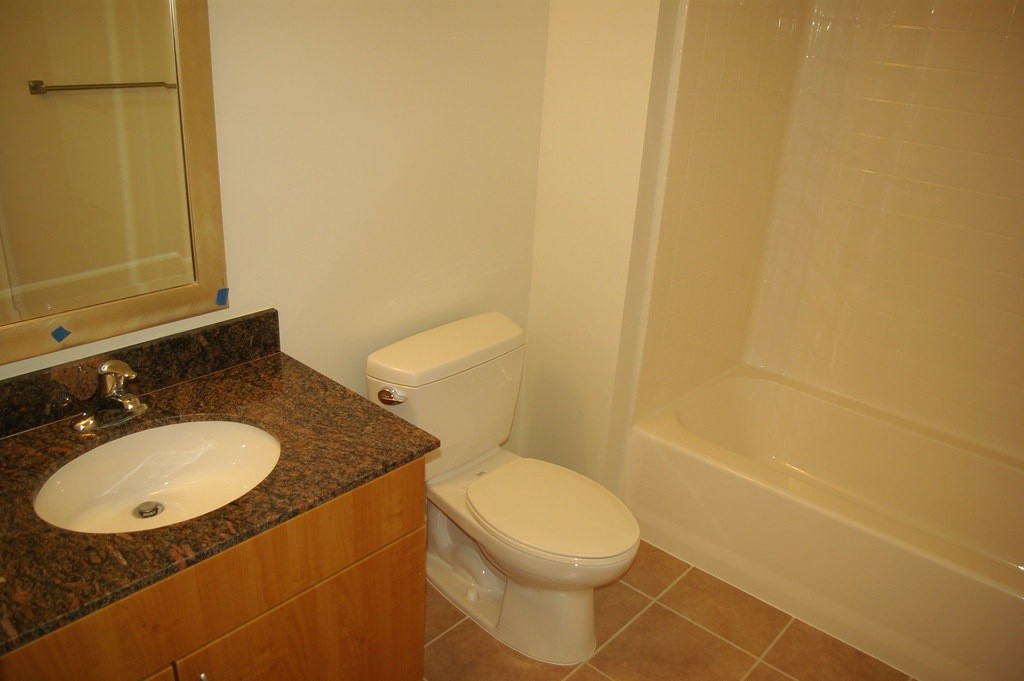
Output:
[66,359,150,442]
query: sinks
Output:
[31,417,284,537]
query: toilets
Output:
[364,310,643,667]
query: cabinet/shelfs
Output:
[0,457,425,680]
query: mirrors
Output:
[0,0,229,365]
